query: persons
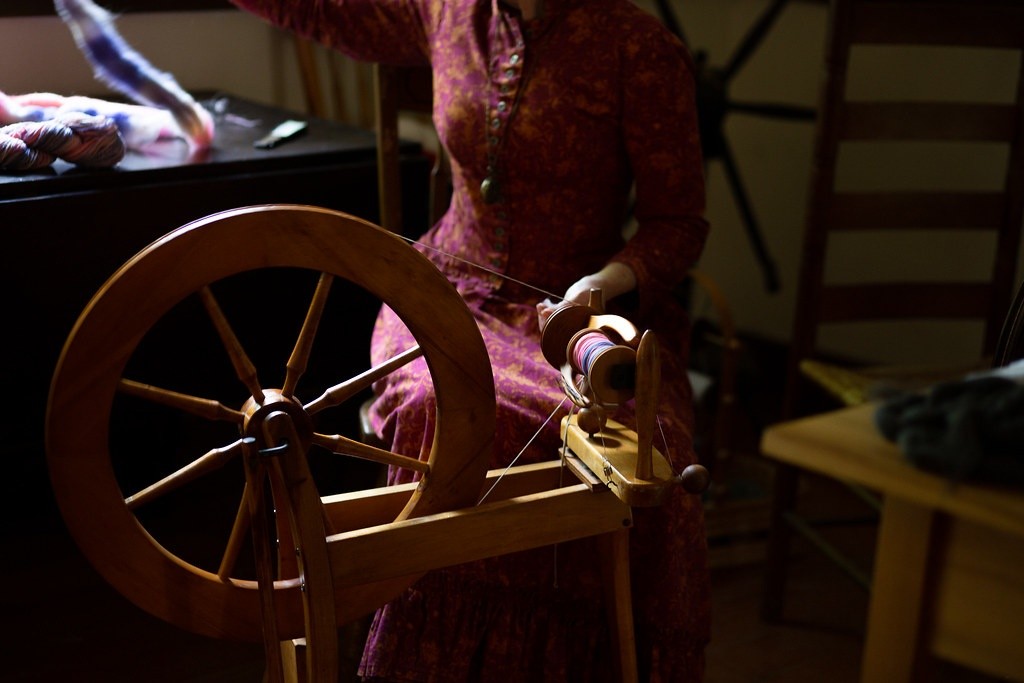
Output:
[235,0,717,682]
